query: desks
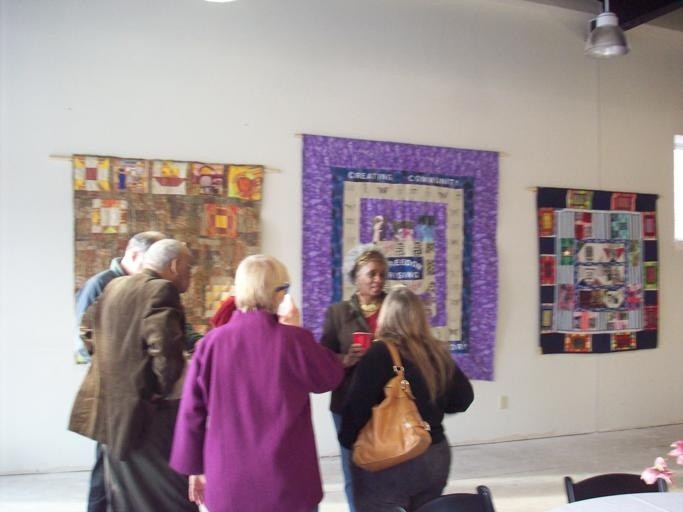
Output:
[547,488,682,512]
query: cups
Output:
[350,332,373,354]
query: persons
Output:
[317,247,392,512]
[328,282,497,512]
[165,251,349,512]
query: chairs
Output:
[393,483,495,511]
[562,468,669,505]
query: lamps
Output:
[583,1,630,60]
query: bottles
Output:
[415,213,436,242]
[393,220,416,242]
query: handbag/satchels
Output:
[353,375,433,473]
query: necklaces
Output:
[356,290,383,313]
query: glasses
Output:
[274,283,289,294]
